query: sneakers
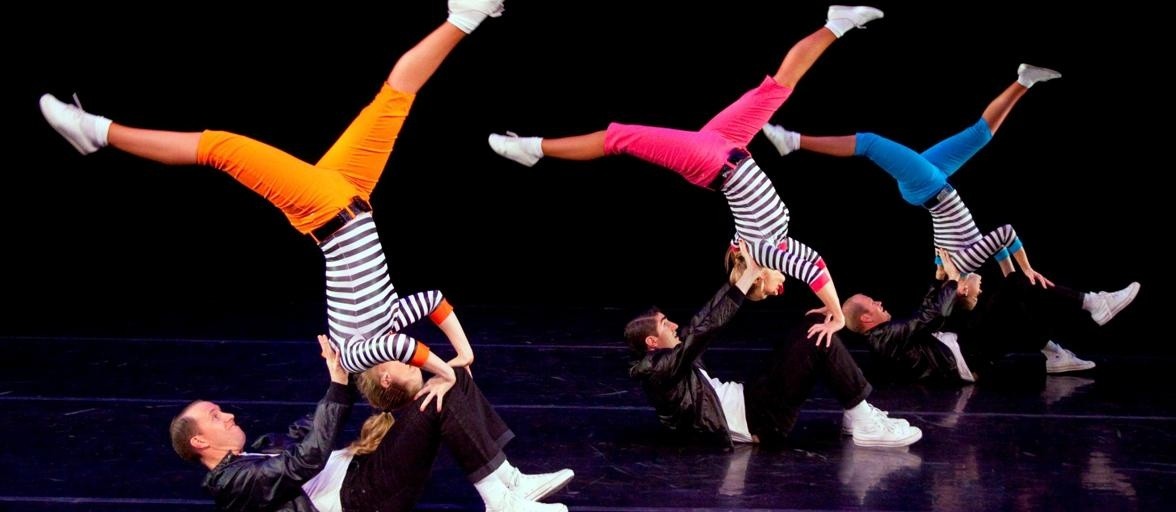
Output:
[1091,281,1142,326]
[1018,64,1061,85]
[828,5,884,28]
[40,94,103,156]
[485,468,575,511]
[1046,349,1096,372]
[763,123,795,157]
[843,404,929,446]
[449,1,506,17]
[488,133,544,166]
[847,439,925,482]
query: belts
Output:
[303,195,372,245]
[923,184,953,209]
[709,148,749,190]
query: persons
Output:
[625,237,925,449]
[485,2,888,351]
[841,245,1142,385]
[37,0,509,413]
[762,62,1063,310]
[170,331,575,512]
